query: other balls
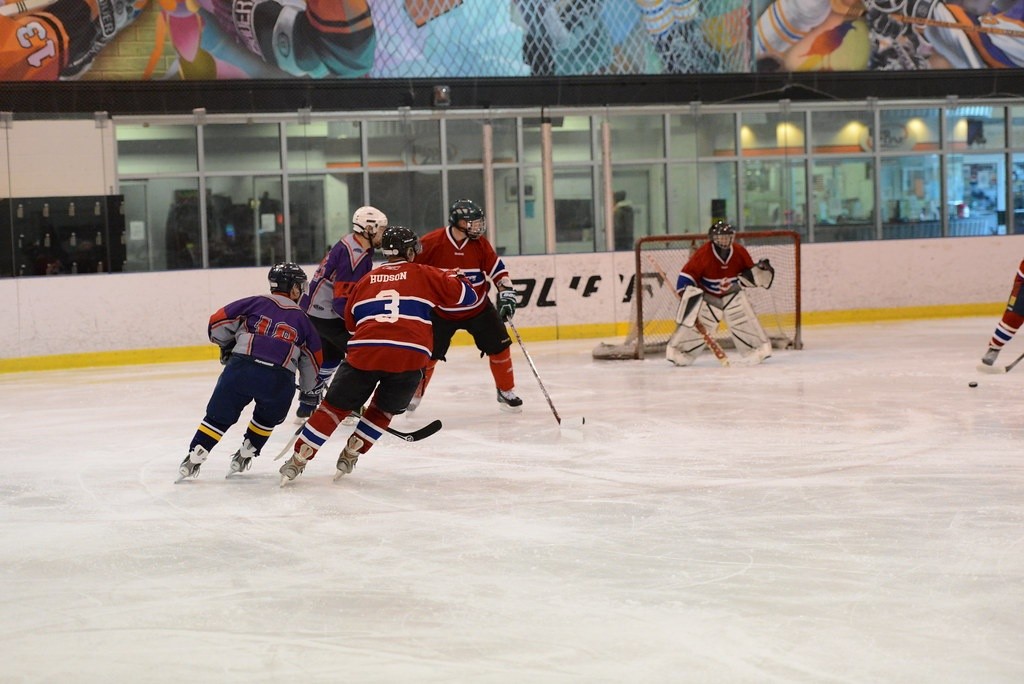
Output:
[969,382,978,387]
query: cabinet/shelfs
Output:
[0,194,128,278]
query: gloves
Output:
[219,338,238,365]
[497,290,518,322]
[298,380,324,406]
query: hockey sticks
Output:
[648,255,772,368]
[296,384,442,443]
[274,420,308,462]
[976,353,1024,375]
[507,314,586,427]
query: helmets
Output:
[380,225,423,257]
[447,199,487,239]
[269,262,308,294]
[352,205,388,233]
[708,221,736,251]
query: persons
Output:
[176,205,480,488]
[666,220,775,366]
[982,260,1024,367]
[599,190,633,251]
[409,200,524,412]
[921,178,995,220]
[0,1,1023,82]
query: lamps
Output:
[433,85,451,107]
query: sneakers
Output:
[981,348,1001,369]
[496,389,523,414]
[225,438,257,479]
[279,444,313,490]
[406,396,422,418]
[332,436,364,483]
[174,444,209,485]
[342,414,359,425]
[294,403,317,425]
